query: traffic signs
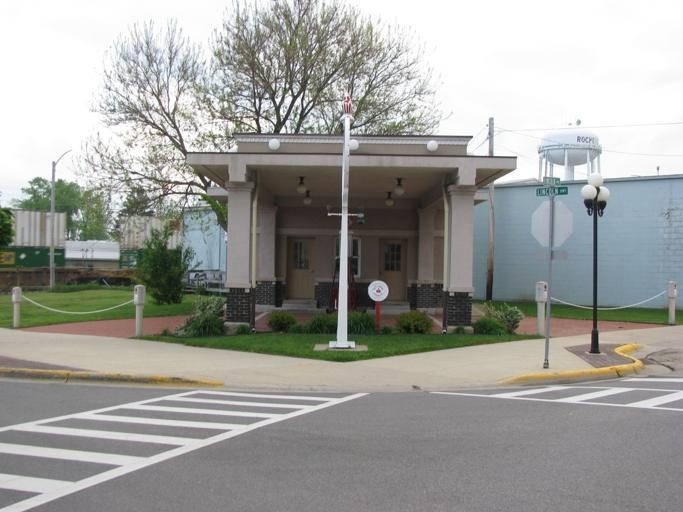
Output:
[536,186,569,196]
[542,177,562,186]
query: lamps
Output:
[427,141,438,152]
[303,191,312,205]
[269,139,280,150]
[350,139,359,151]
[296,176,306,194]
[394,177,405,196]
[384,192,394,207]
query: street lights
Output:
[49,150,71,290]
[581,172,609,353]
